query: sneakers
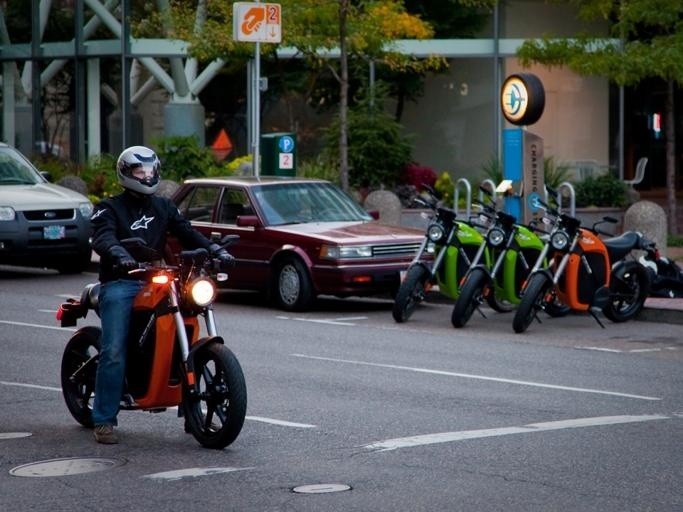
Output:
[94,425,118,445]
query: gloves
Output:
[208,244,237,268]
[109,246,135,270]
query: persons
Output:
[90,146,236,444]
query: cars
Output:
[166,175,434,308]
[0,141,93,274]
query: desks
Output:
[559,164,616,180]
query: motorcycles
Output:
[392,175,517,327]
[451,186,573,325]
[55,232,247,450]
[514,186,658,337]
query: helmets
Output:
[116,146,162,197]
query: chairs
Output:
[618,157,647,190]
[573,159,597,182]
